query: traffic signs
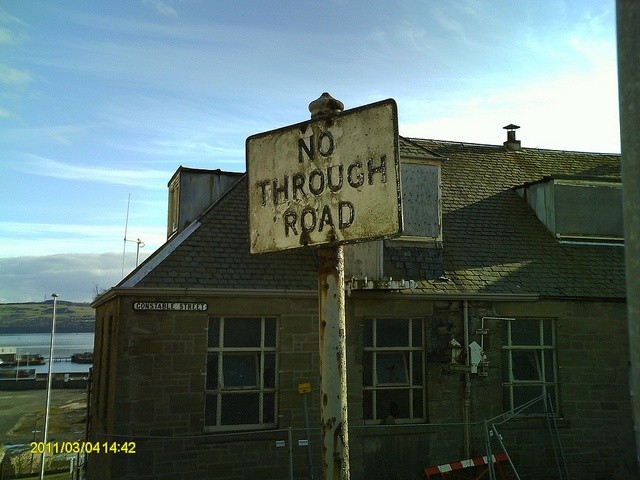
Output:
[237,92,409,258]
